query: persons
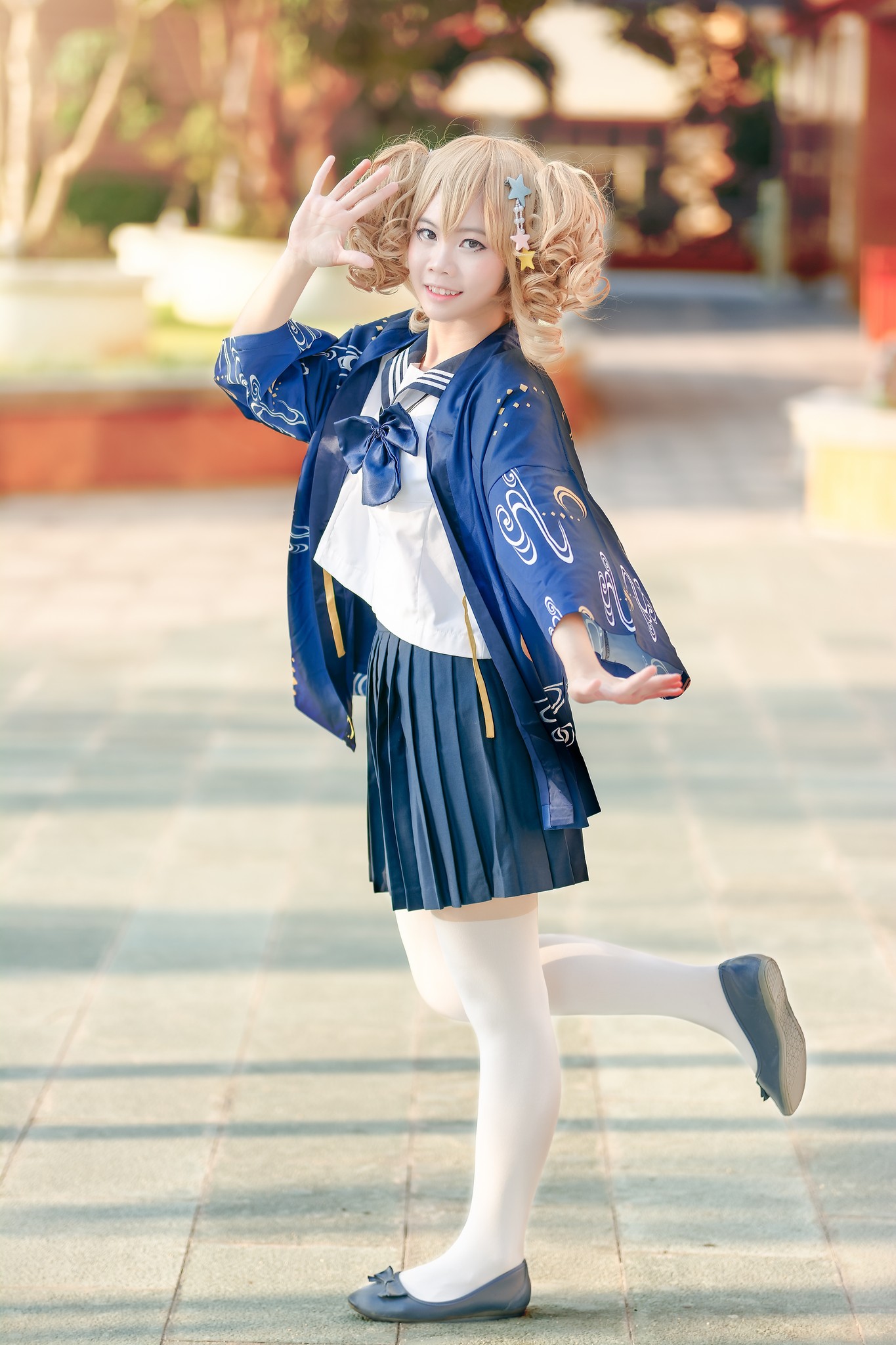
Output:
[214,137,809,1321]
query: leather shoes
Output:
[719,954,805,1117]
[347,1258,532,1322]
